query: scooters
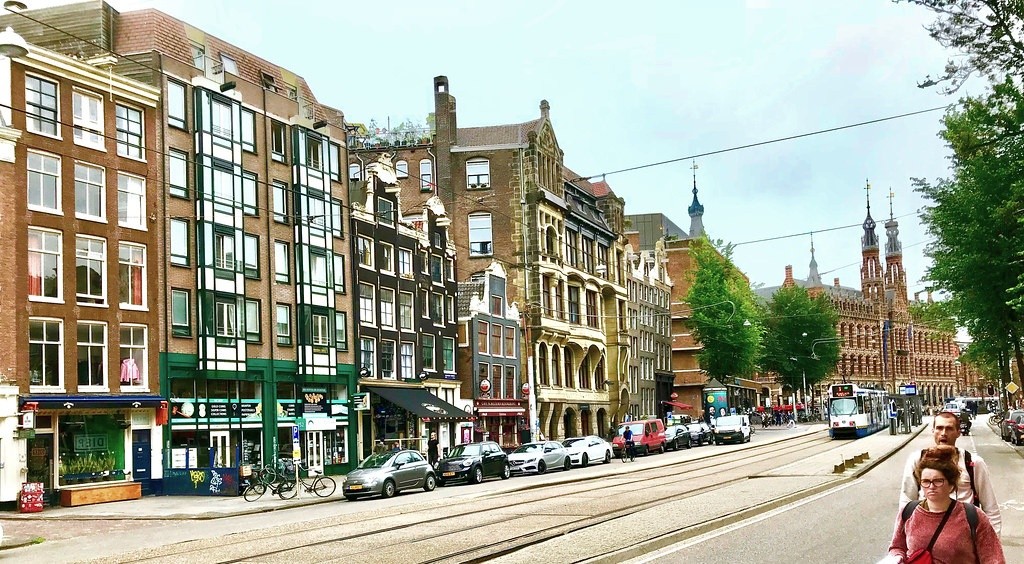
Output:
[959,421,970,436]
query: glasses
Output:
[919,477,948,487]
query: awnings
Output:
[368,388,475,422]
[666,401,694,410]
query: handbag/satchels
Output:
[904,549,933,564]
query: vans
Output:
[611,419,667,457]
[714,415,752,445]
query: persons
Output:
[680,415,691,424]
[427,432,441,466]
[960,409,972,428]
[708,406,726,416]
[736,404,797,429]
[620,426,634,458]
[373,437,400,454]
[879,412,1006,564]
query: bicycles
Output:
[620,440,635,463]
[242,460,337,503]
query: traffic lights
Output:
[988,385,994,395]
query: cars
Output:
[506,440,572,476]
[560,435,613,468]
[436,441,511,484]
[943,403,961,417]
[685,422,714,447]
[342,448,438,502]
[999,409,1024,446]
[663,425,692,450]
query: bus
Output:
[827,383,890,440]
[954,396,1000,414]
